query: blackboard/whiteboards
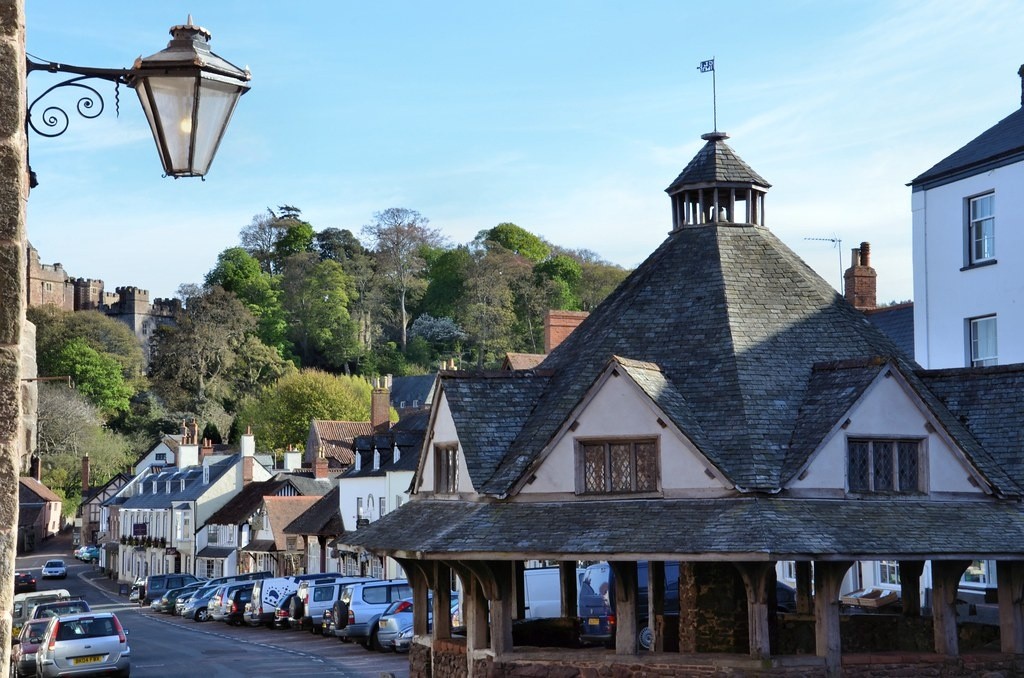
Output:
[119,584,129,594]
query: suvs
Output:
[577,560,815,652]
[12,588,94,643]
[128,561,563,654]
[31,610,132,678]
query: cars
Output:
[12,614,87,678]
[14,570,37,594]
[72,544,100,564]
[41,559,68,580]
